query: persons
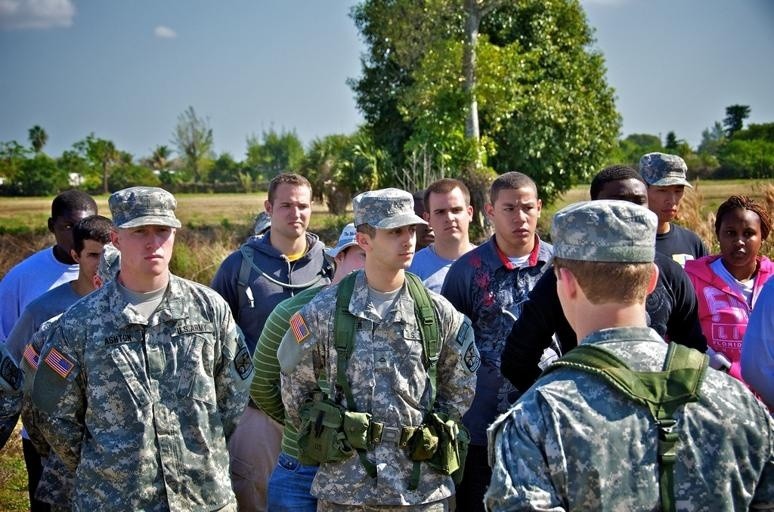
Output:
[1,147,774,512]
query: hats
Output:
[353,187,429,230]
[108,186,182,228]
[254,212,271,234]
[639,152,693,188]
[96,242,121,283]
[540,199,658,274]
[324,222,358,258]
